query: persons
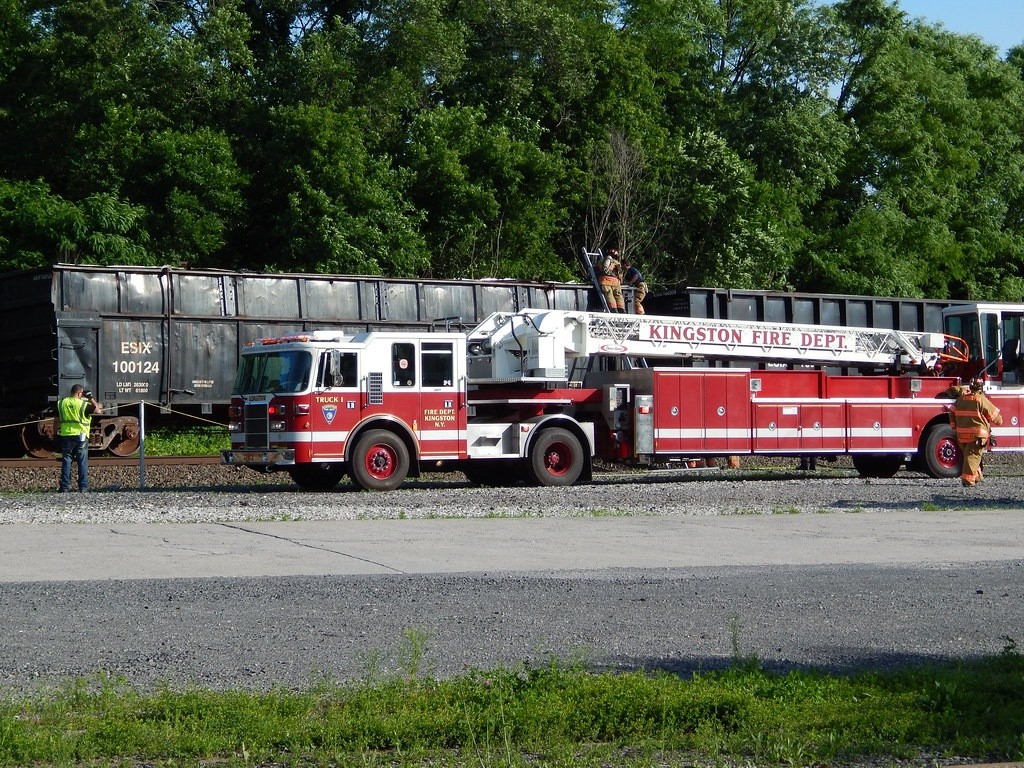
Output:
[796,457,818,471]
[621,259,648,315]
[53,384,101,493]
[596,248,627,313]
[949,378,1002,486]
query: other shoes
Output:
[611,309,619,313]
[620,308,627,313]
[58,489,67,493]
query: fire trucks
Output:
[220,307,971,487]
[942,303,1024,454]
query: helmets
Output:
[970,377,984,389]
[607,249,619,258]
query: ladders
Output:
[581,247,690,469]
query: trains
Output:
[0,262,984,460]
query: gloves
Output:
[84,390,92,399]
